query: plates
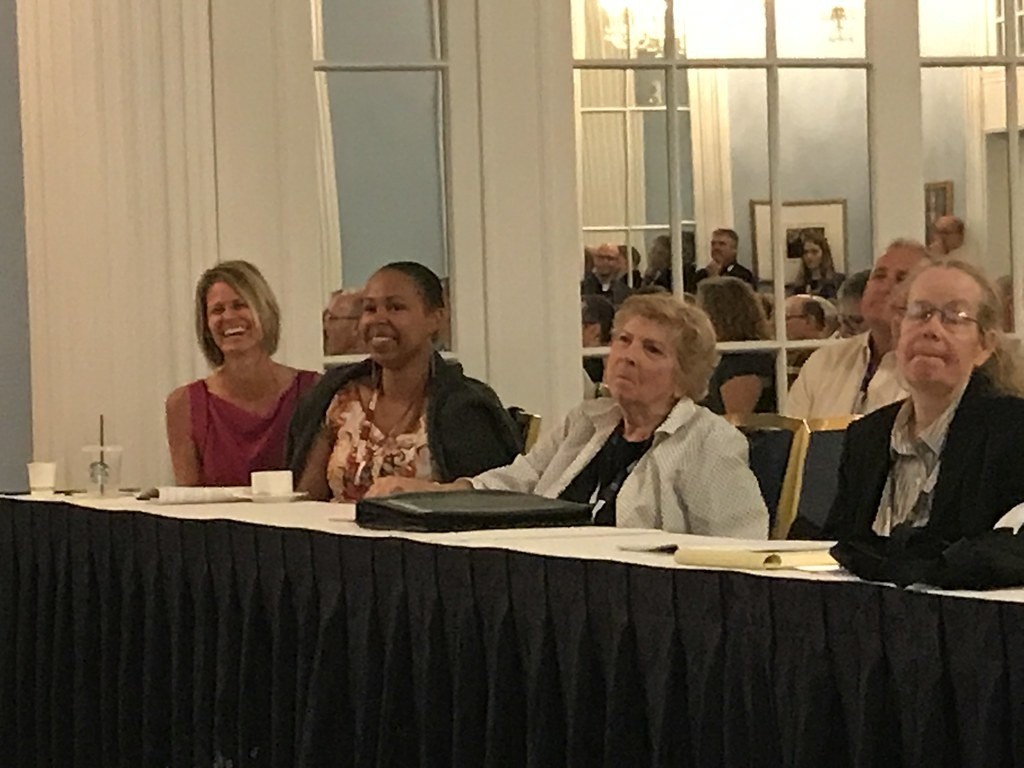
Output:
[235,493,309,503]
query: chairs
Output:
[723,413,863,541]
[507,407,541,454]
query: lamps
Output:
[600,0,685,55]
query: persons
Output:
[783,239,936,425]
[929,217,978,266]
[794,230,845,299]
[287,260,521,502]
[827,261,1022,540]
[167,259,324,487]
[700,277,779,416]
[580,227,756,382]
[364,289,770,543]
[760,267,870,391]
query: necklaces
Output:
[351,381,417,485]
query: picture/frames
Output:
[925,181,953,246]
[748,198,849,285]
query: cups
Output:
[27,463,55,497]
[82,447,121,497]
[251,470,293,496]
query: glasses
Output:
[597,254,619,261]
[711,240,733,246]
[322,311,360,323]
[785,312,810,320]
[902,307,986,335]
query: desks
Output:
[2,491,1024,768]
